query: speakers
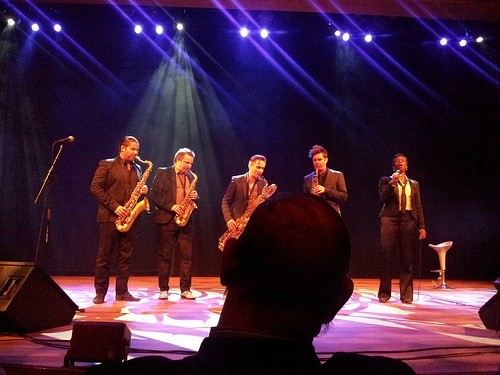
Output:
[71,321,132,363]
[477,292,500,331]
[0,262,79,334]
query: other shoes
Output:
[402,299,412,304]
[379,297,387,303]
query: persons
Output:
[89,136,148,304]
[83,191,418,375]
[377,154,425,303]
[150,146,199,300]
[304,145,349,219]
[221,154,269,230]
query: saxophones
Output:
[174,168,198,227]
[115,155,153,232]
[216,173,277,252]
[312,166,320,197]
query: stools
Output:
[428,241,455,290]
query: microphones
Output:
[54,136,75,144]
[395,170,400,182]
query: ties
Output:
[398,179,407,213]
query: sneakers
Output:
[159,291,168,300]
[181,291,196,300]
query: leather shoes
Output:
[93,293,105,303]
[116,291,141,302]
